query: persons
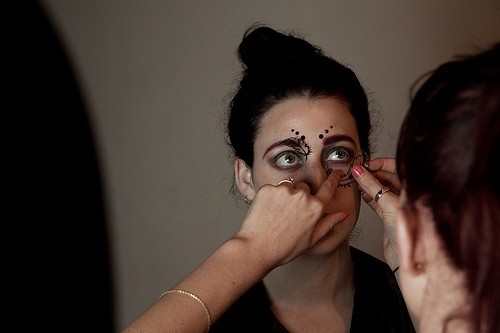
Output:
[203,22,416,333]
[119,40,500,333]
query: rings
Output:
[276,176,297,190]
[374,185,392,204]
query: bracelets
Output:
[156,289,212,331]
[391,262,401,277]
